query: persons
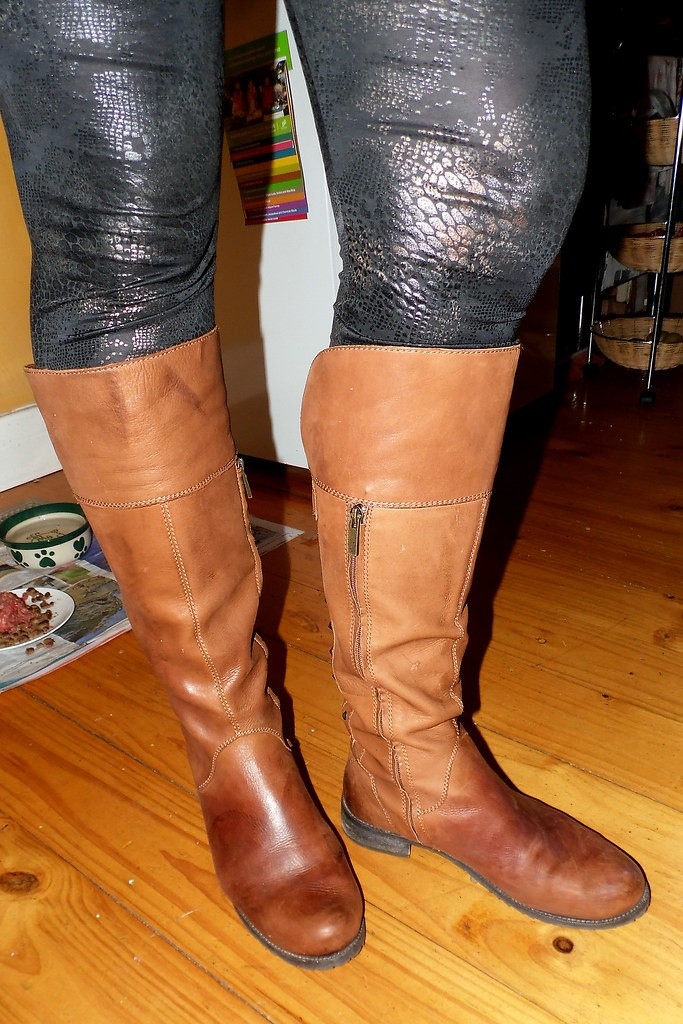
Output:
[1,0,652,968]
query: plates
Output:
[0,588,75,651]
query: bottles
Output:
[612,263,629,304]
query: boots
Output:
[298,339,652,927]
[24,323,367,969]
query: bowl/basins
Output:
[0,503,91,569]
